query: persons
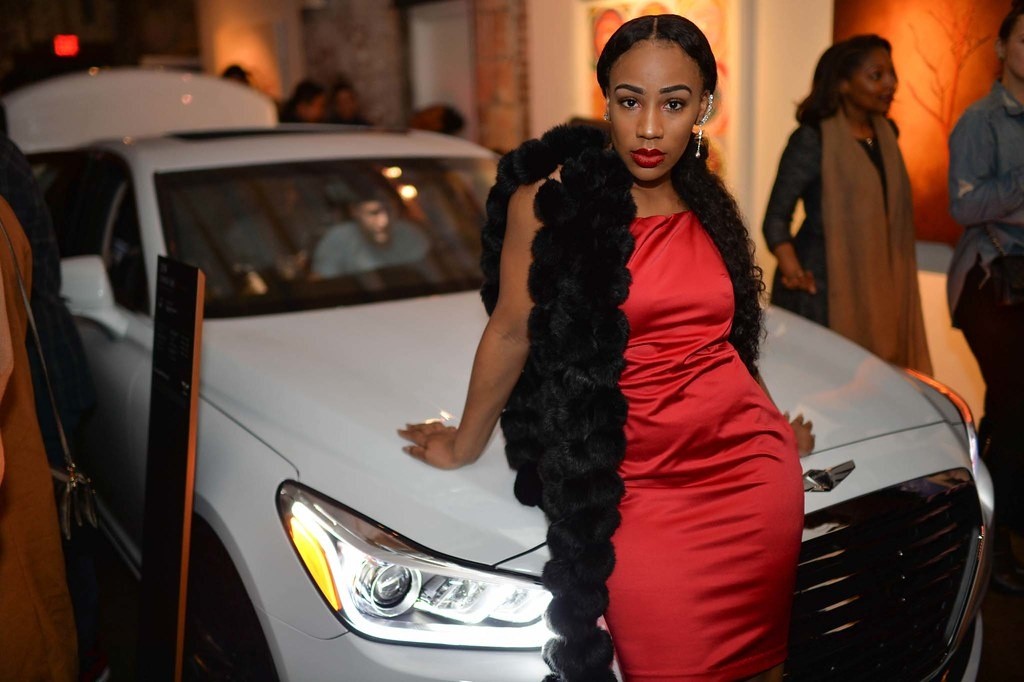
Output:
[944,4,1024,595]
[310,189,431,280]
[325,72,375,128]
[278,76,328,124]
[218,64,251,85]
[401,14,815,682]
[762,33,936,381]
[407,103,467,137]
[0,100,109,682]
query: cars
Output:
[2,66,1000,682]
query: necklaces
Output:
[865,136,874,149]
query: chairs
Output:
[141,190,459,315]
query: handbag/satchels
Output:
[47,462,103,580]
